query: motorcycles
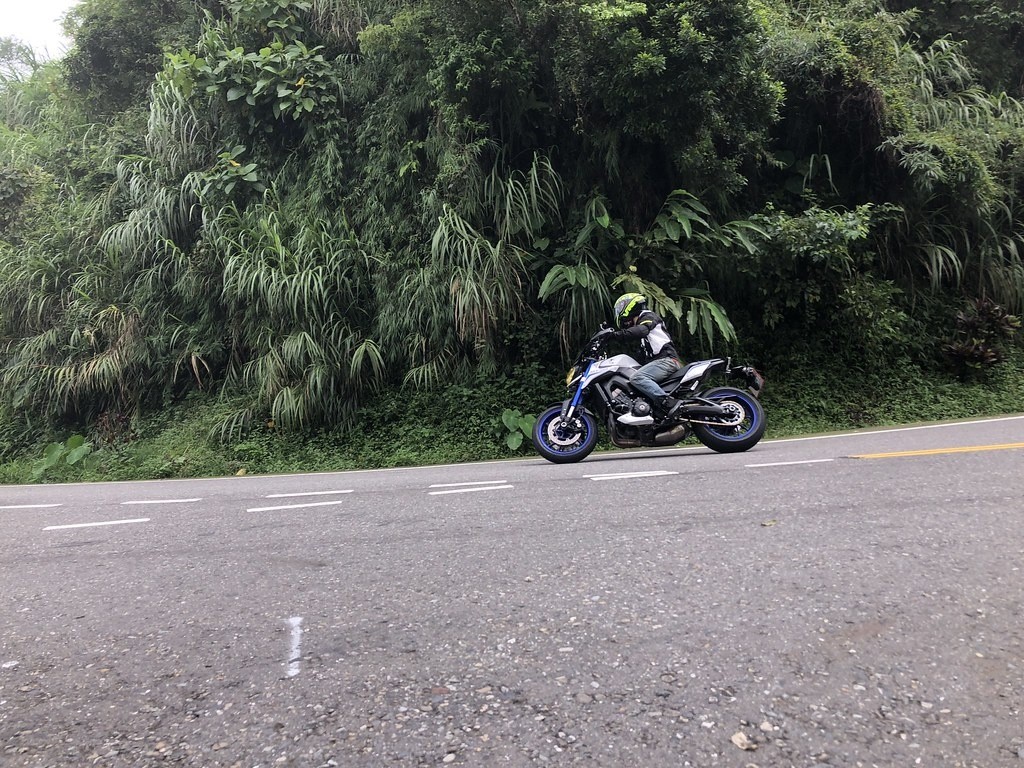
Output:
[532,321,767,464]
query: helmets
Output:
[613,293,647,330]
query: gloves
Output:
[606,332,617,342]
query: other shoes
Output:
[659,396,685,417]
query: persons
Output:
[602,293,683,417]
